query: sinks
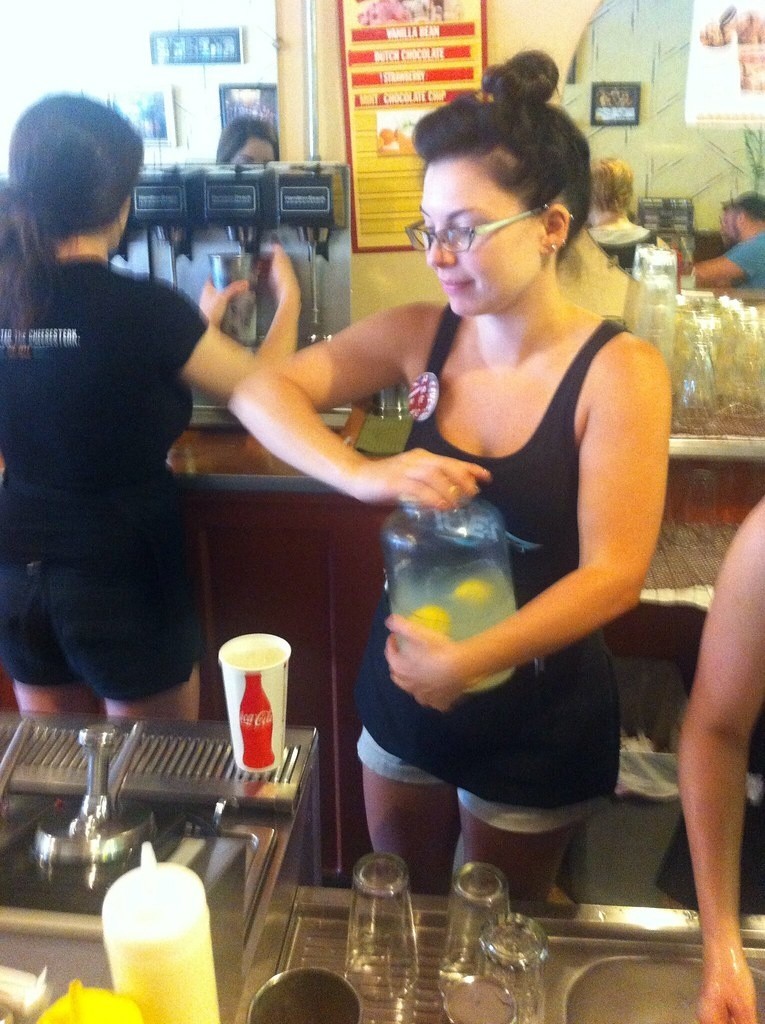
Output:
[0,783,278,943]
[560,954,765,1023]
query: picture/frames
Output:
[108,78,178,148]
[590,80,641,127]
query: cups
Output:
[245,966,365,1024]
[480,912,551,1023]
[738,42,765,99]
[209,250,263,347]
[372,385,413,423]
[631,236,765,436]
[437,861,512,1000]
[217,632,292,774]
[345,851,423,1001]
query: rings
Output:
[448,486,457,494]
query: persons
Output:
[228,46,671,899]
[658,493,765,1024]
[695,192,765,288]
[584,158,671,272]
[599,88,636,107]
[0,96,301,721]
[216,115,279,164]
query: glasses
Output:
[406,204,576,252]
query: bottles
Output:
[382,490,518,691]
[33,977,143,1023]
[103,841,221,1023]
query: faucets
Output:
[70,714,159,823]
